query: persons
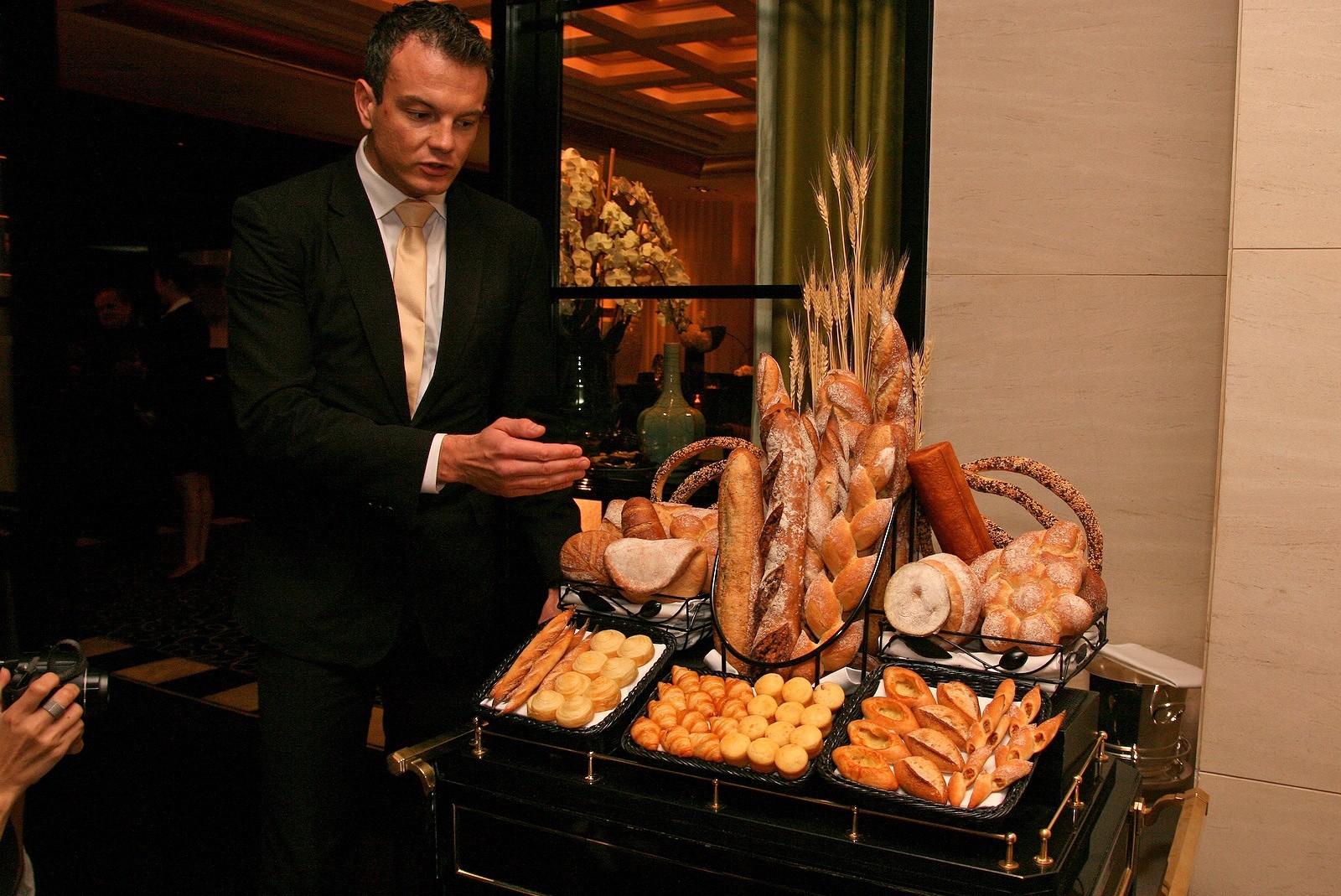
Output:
[228,0,591,895]
[140,253,213,583]
[0,666,84,896]
[63,288,157,540]
[636,353,664,405]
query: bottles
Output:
[637,342,706,472]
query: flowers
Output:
[556,143,721,357]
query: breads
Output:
[714,310,916,685]
[629,665,845,781]
[487,608,655,728]
[559,437,767,605]
[884,454,1109,656]
[829,666,1066,809]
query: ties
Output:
[392,199,435,419]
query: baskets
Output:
[826,661,1054,823]
[617,664,848,787]
[484,608,680,748]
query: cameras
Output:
[0,649,109,720]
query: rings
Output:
[43,698,65,719]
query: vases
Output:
[632,339,709,474]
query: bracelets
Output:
[654,378,659,381]
